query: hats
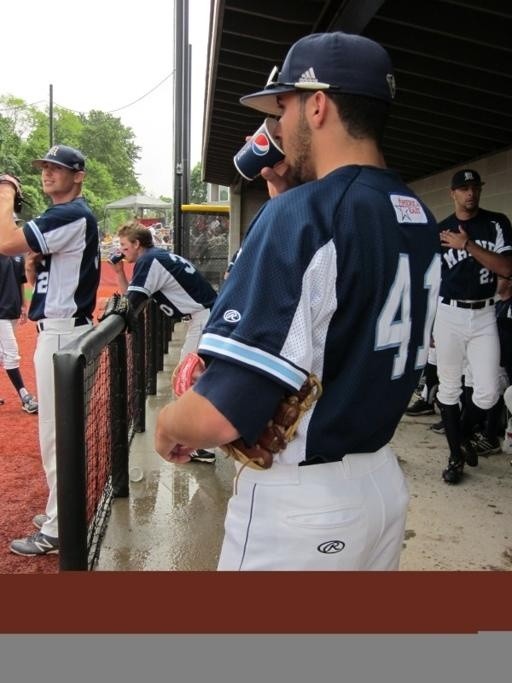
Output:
[238,30,398,115]
[451,169,485,191]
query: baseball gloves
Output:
[1,172,22,212]
[171,340,322,469]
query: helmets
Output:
[31,145,85,172]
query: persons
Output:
[0,145,102,556]
[153,31,442,573]
[404,169,512,485]
[0,209,38,415]
[111,221,217,465]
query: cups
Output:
[108,248,127,264]
[129,463,144,481]
[234,119,284,183]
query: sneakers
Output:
[188,448,216,465]
[405,384,511,485]
[9,512,60,557]
[22,393,38,414]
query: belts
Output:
[442,297,495,309]
